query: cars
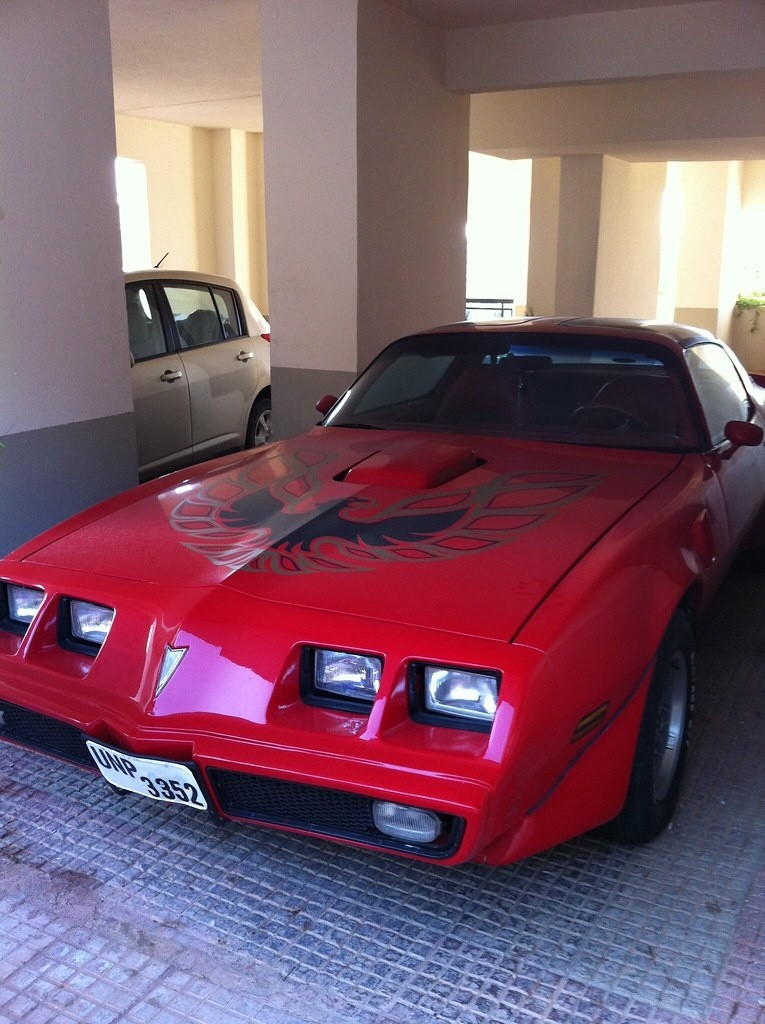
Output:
[0,317,765,869]
[124,253,272,485]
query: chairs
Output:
[433,360,535,429]
[585,369,688,437]
[181,309,222,346]
